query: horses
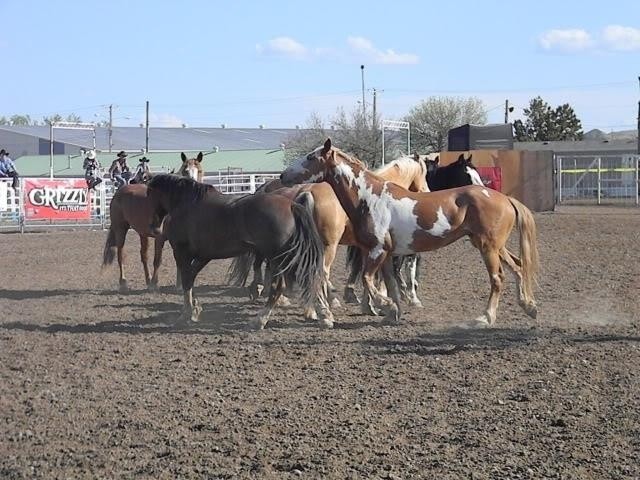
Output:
[228,138,485,319]
[139,168,336,329]
[273,140,547,326]
[100,151,204,287]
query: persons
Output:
[109,151,130,190]
[137,157,151,181]
[83,150,102,190]
[0,149,19,190]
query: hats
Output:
[1,148,10,156]
[117,151,128,158]
[86,150,95,159]
[139,158,149,162]
